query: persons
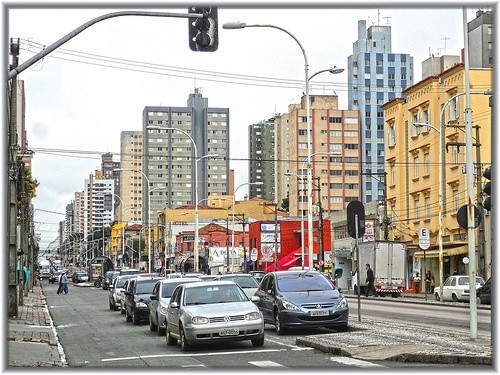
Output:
[424,269,431,293]
[365,264,378,298]
[414,272,421,294]
[57,271,70,295]
[431,276,435,293]
[49,269,55,285]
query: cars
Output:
[39,254,88,284]
[164,281,266,352]
[433,276,486,302]
[476,277,491,304]
[250,272,350,335]
[101,270,269,336]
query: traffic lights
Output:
[483,167,491,212]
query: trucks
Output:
[350,240,406,298]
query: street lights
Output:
[284,173,313,271]
[412,89,491,301]
[222,22,344,269]
[113,169,165,273]
[64,192,135,267]
[146,126,219,273]
[301,152,342,269]
[232,182,264,274]
